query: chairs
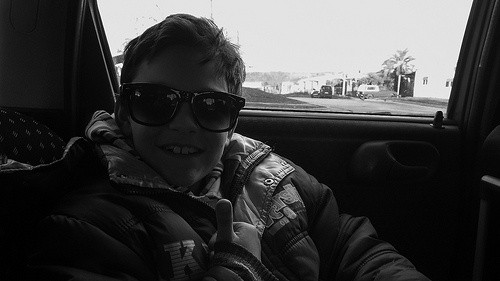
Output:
[0,106,66,166]
[470,125,500,281]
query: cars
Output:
[310,90,319,98]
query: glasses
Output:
[120,82,245,133]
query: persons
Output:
[37,13,433,280]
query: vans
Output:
[320,85,333,98]
